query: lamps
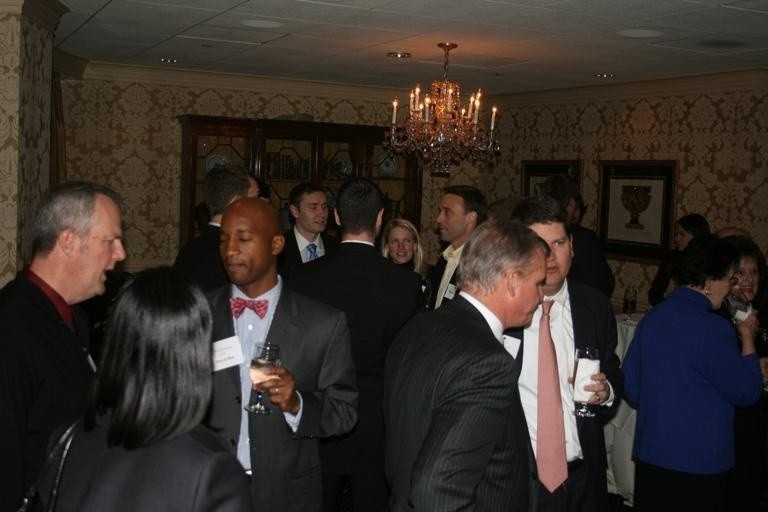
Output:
[389,40,501,178]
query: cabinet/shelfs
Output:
[177,113,423,256]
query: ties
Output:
[305,244,320,262]
[535,297,571,494]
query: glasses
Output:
[721,276,739,286]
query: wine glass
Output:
[243,343,280,416]
[573,346,599,419]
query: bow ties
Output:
[229,296,269,322]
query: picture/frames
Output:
[520,159,584,207]
[597,159,678,265]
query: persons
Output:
[622,232,764,511]
[274,183,337,270]
[708,234,768,512]
[381,220,426,279]
[506,194,624,512]
[647,214,711,308]
[381,219,552,511]
[172,162,260,300]
[35,262,253,511]
[195,197,359,511]
[0,180,129,512]
[431,182,615,311]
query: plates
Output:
[332,151,400,176]
[275,114,313,121]
[204,154,232,173]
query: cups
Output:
[725,289,750,319]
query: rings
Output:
[595,393,600,401]
[275,387,279,393]
